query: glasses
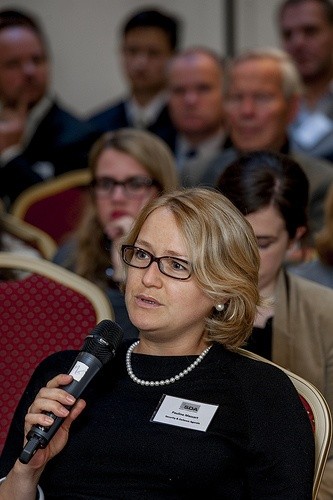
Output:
[122,245,193,280]
[87,178,164,198]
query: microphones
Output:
[19,319,124,465]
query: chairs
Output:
[0,253,115,456]
[14,171,96,251]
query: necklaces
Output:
[125,341,212,385]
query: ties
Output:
[177,148,196,184]
[134,110,150,129]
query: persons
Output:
[0,188,315,499]
[0,0,333,500]
